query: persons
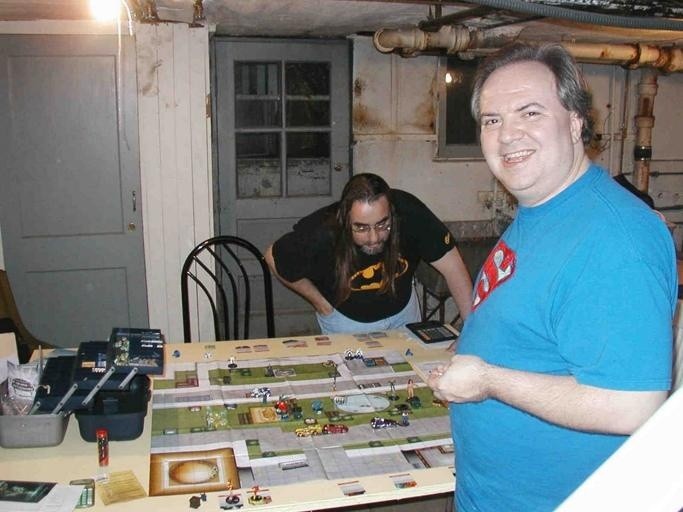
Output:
[263,173,472,334]
[427,41,679,512]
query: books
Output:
[0,469,147,512]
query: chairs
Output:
[180,235,274,342]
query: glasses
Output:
[354,224,391,232]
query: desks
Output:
[1,328,520,512]
